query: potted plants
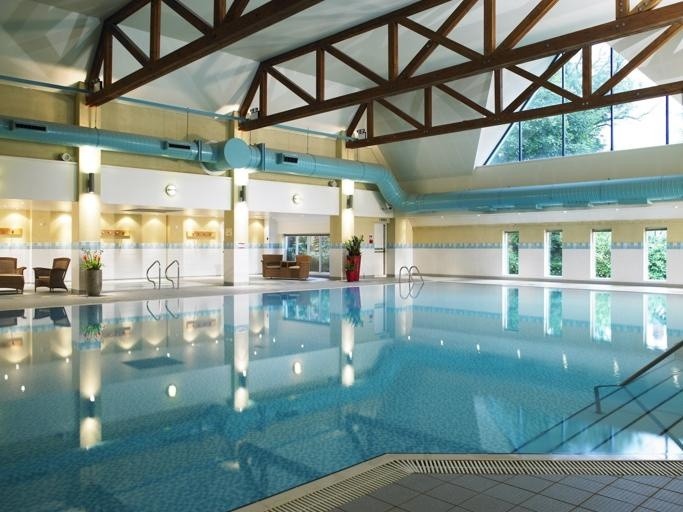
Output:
[341,234,366,281]
[342,287,364,328]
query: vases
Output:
[84,269,103,296]
[79,303,102,325]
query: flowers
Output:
[78,323,105,343]
[79,247,105,274]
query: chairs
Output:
[262,293,284,306]
[0,256,71,294]
[0,307,72,328]
[260,253,312,282]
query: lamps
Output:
[239,185,247,202]
[347,194,353,208]
[238,373,247,388]
[87,173,95,193]
[88,397,96,418]
[347,353,354,365]
